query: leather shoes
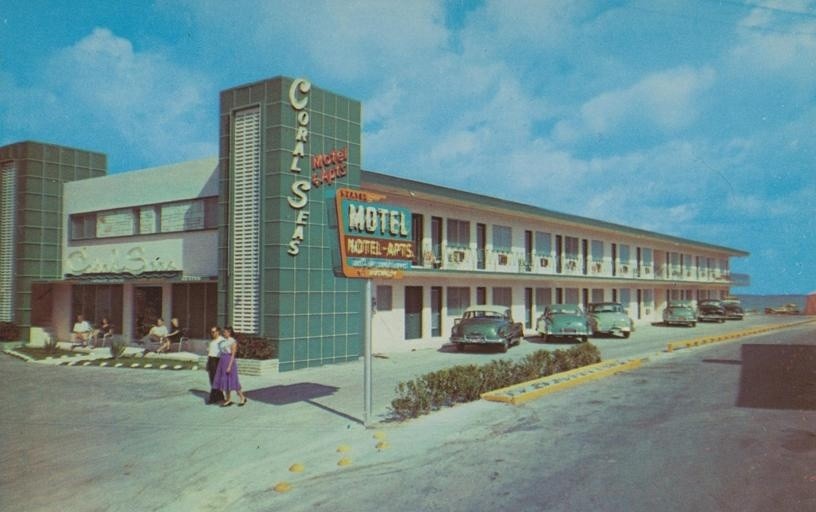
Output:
[222,400,233,407]
[208,396,224,404]
[238,398,247,406]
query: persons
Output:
[91,317,113,349]
[211,326,248,407]
[142,317,168,352]
[155,317,183,353]
[205,326,225,405]
[71,314,92,348]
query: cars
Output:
[587,301,636,338]
[662,298,744,328]
[535,303,597,344]
[450,303,524,353]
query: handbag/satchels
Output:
[206,360,210,371]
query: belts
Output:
[208,357,219,360]
